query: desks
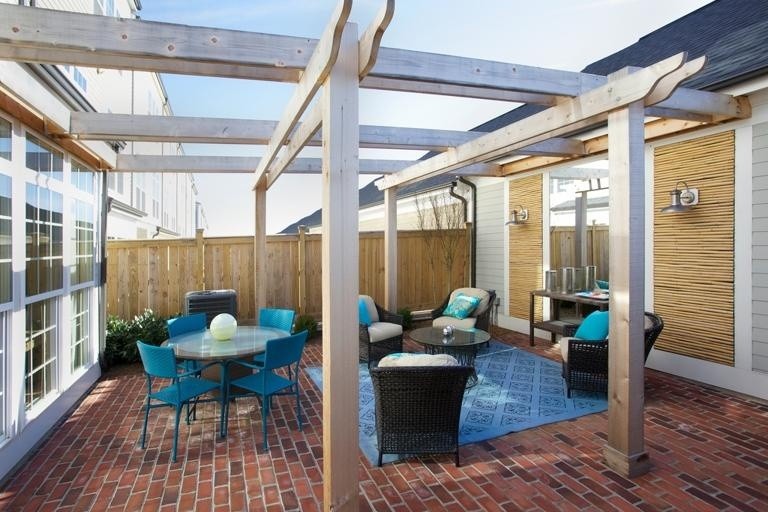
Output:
[526,288,609,346]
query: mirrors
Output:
[543,157,610,288]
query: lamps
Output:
[659,179,700,214]
[504,204,530,227]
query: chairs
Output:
[432,287,497,352]
[139,307,309,462]
[358,292,404,369]
[557,310,665,399]
[366,350,475,467]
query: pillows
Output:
[575,309,609,342]
[359,298,373,327]
[442,293,485,321]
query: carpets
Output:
[304,339,608,465]
